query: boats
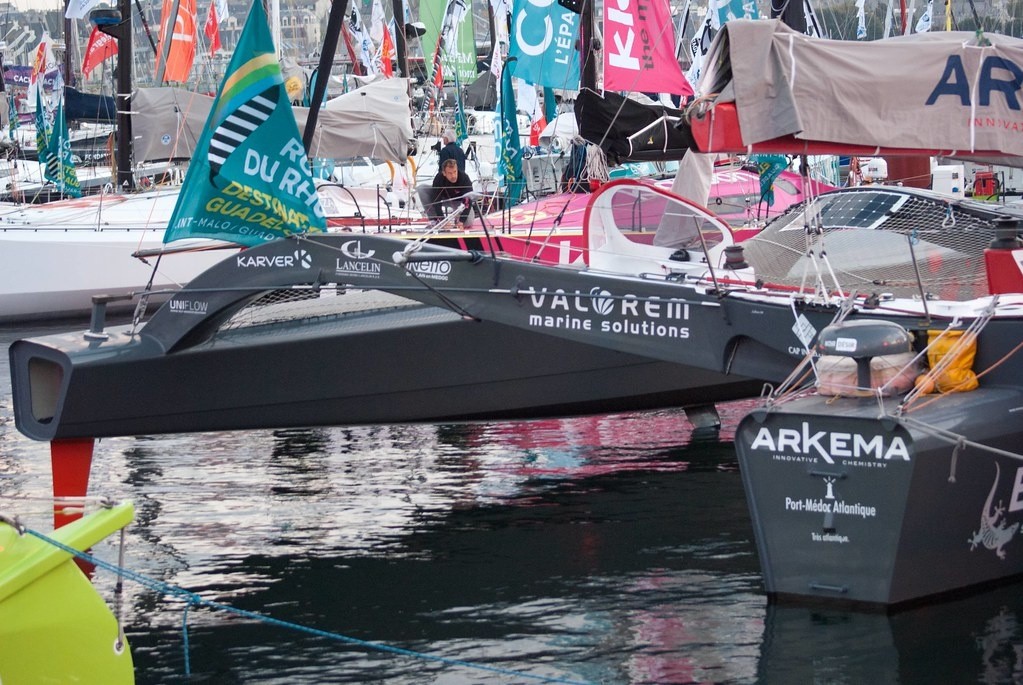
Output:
[1,1,1023,685]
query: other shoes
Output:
[426,224,445,229]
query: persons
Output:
[430,159,473,229]
[438,129,465,174]
